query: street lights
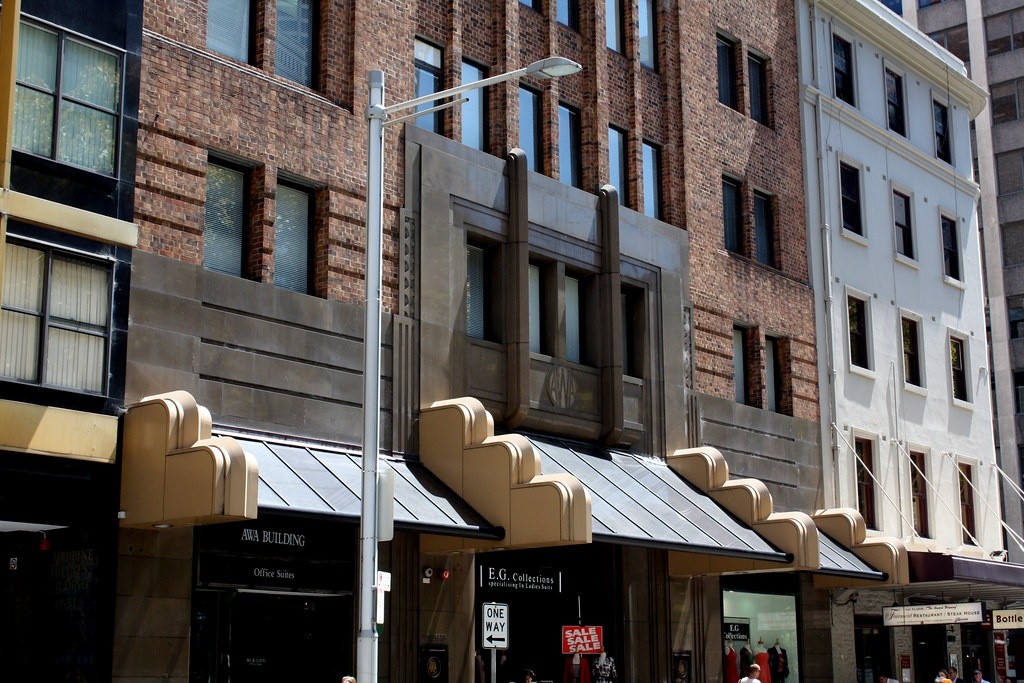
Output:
[356,56,585,682]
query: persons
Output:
[737,664,762,683]
[973,670,990,683]
[739,643,754,678]
[935,670,950,683]
[948,667,965,683]
[592,651,618,683]
[725,640,738,683]
[767,642,790,683]
[564,653,590,683]
[878,674,899,683]
[752,643,771,683]
[342,676,356,683]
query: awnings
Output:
[204,430,508,539]
[497,434,796,563]
[816,523,888,580]
